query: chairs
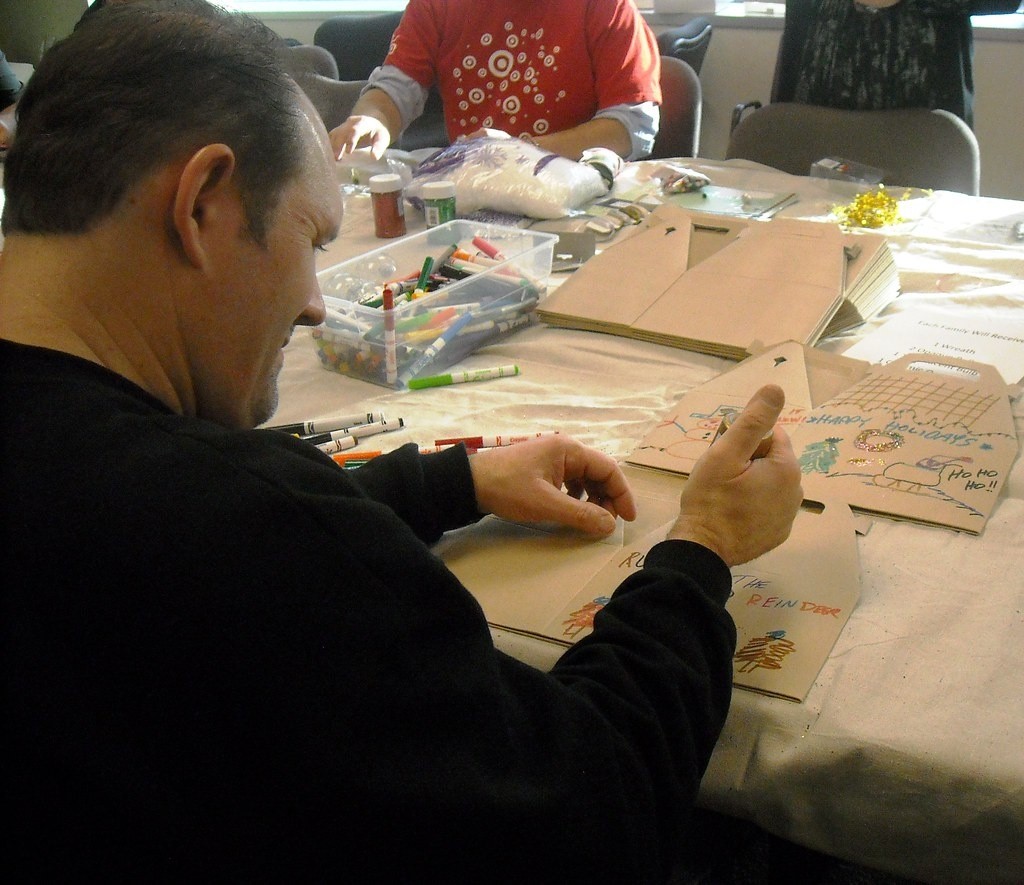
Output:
[275,45,339,81]
[656,16,712,76]
[425,56,703,159]
[725,101,982,196]
[291,71,402,149]
[313,10,439,116]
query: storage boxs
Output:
[305,219,559,391]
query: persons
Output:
[770,0,1021,130]
[3,1,804,878]
[329,1,663,162]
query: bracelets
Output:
[518,132,539,148]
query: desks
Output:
[258,158,1024,885]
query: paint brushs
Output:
[263,409,559,469]
[314,235,544,387]
[408,363,518,390]
